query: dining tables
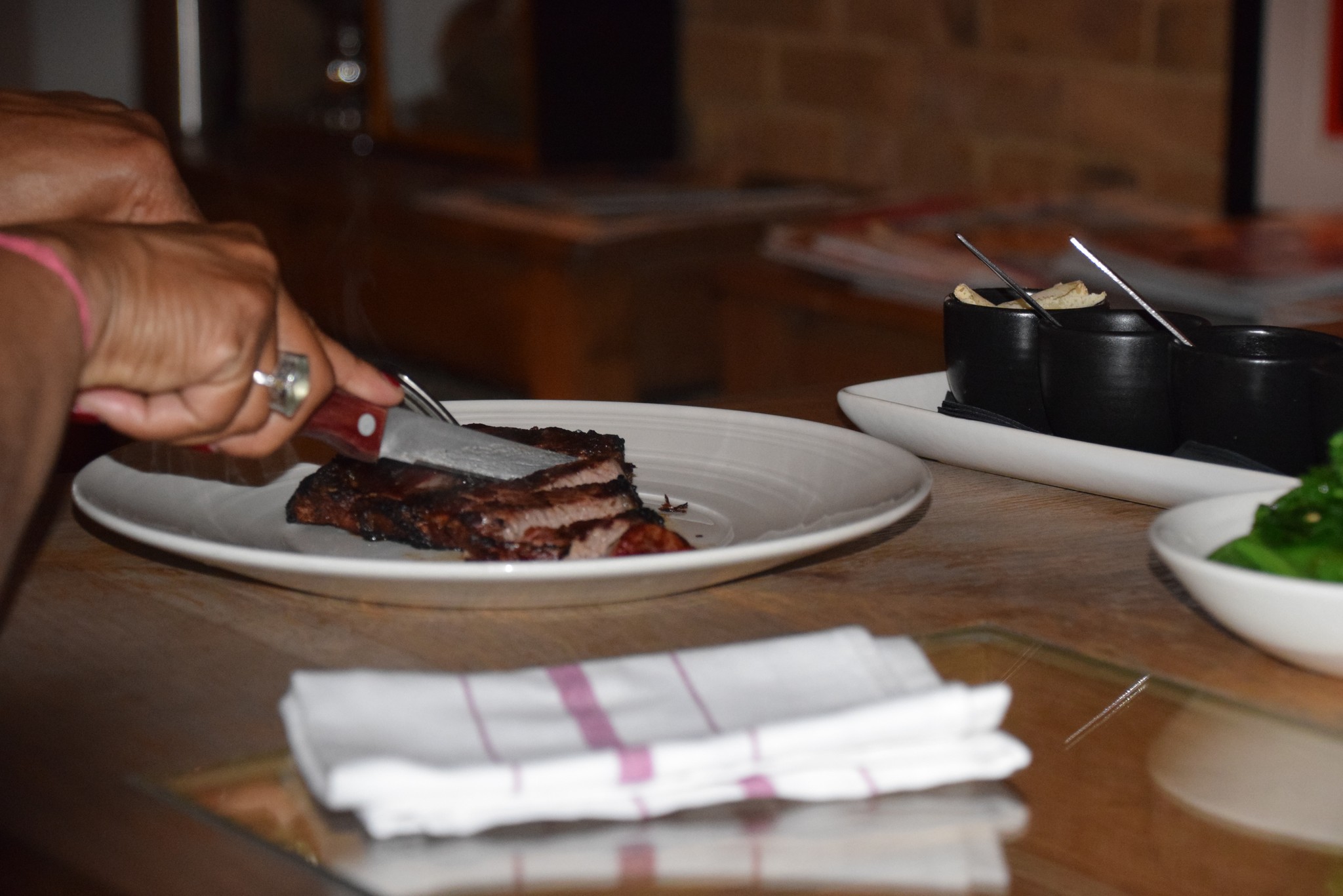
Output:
[0,454,1343,896]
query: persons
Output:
[0,75,408,585]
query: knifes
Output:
[297,388,581,481]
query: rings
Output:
[263,351,311,419]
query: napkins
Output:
[279,626,1032,841]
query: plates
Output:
[70,399,932,609]
[835,371,1305,507]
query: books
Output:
[755,186,1343,324]
[414,178,896,244]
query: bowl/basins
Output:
[1148,490,1342,675]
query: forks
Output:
[395,372,460,426]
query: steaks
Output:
[285,423,697,563]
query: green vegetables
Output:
[1205,432,1343,587]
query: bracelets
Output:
[0,231,95,352]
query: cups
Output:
[1170,324,1343,476]
[1037,306,1213,456]
[943,289,1110,435]
